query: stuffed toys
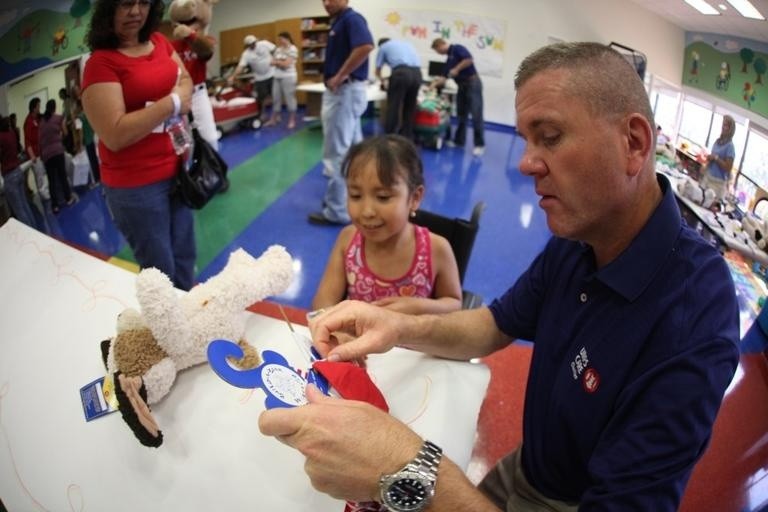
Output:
[98,244,294,447]
[167,0,231,194]
[677,178,722,213]
[742,214,768,249]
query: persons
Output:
[24,97,54,204]
[308,0,375,226]
[655,126,671,145]
[375,38,422,138]
[311,133,462,316]
[0,113,39,231]
[258,40,741,512]
[8,114,34,197]
[57,89,73,136]
[37,100,80,214]
[706,115,736,181]
[427,37,485,156]
[230,35,277,130]
[262,32,299,129]
[75,101,101,189]
[81,0,197,293]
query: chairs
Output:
[408,199,487,288]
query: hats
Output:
[243,36,257,45]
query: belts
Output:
[393,64,422,71]
[345,77,369,85]
[192,83,208,94]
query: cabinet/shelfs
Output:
[244,21,274,105]
[219,27,244,78]
[299,14,332,106]
[274,18,300,105]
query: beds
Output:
[208,93,261,140]
[414,112,455,150]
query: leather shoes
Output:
[308,215,351,225]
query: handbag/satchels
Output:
[177,109,229,210]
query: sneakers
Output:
[472,146,485,157]
[445,141,461,149]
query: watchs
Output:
[376,440,444,512]
[715,154,720,160]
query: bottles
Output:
[164,115,192,156]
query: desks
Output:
[297,82,387,119]
[654,168,767,264]
[0,214,490,511]
